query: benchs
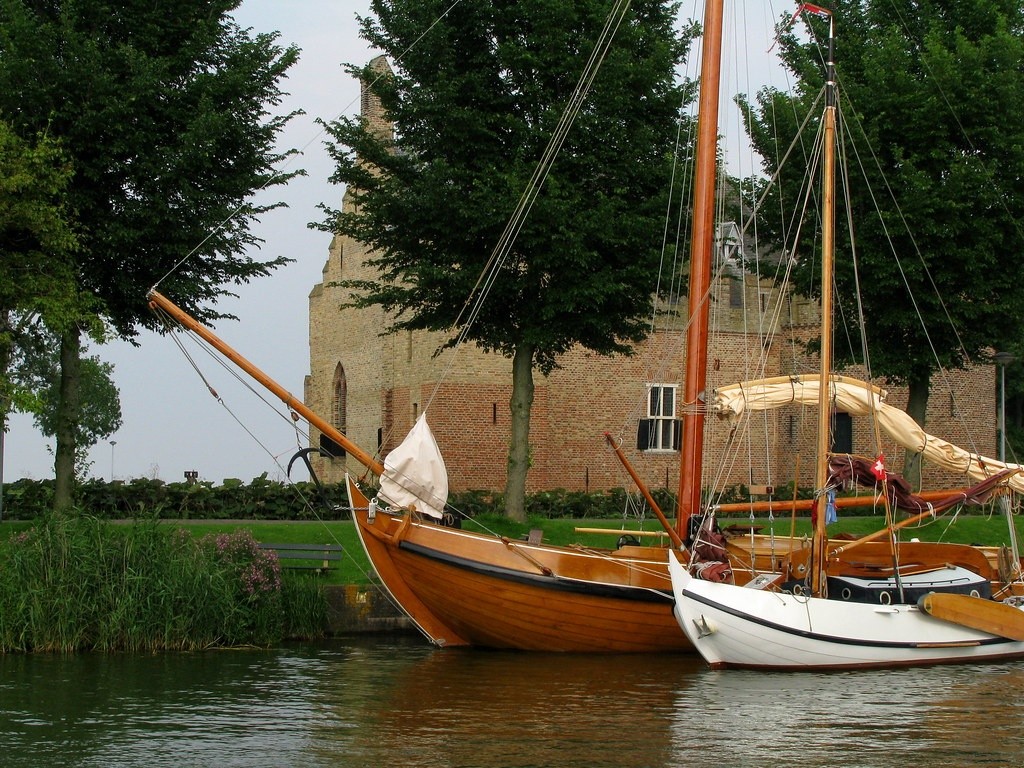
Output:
[257,541,343,577]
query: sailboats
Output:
[142,0,1021,669]
